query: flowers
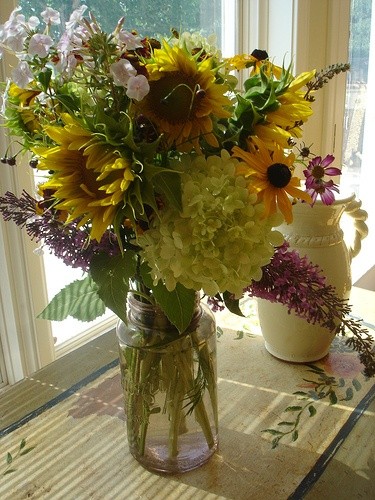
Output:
[0,6,375,476]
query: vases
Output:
[116,292,220,475]
[256,193,369,364]
